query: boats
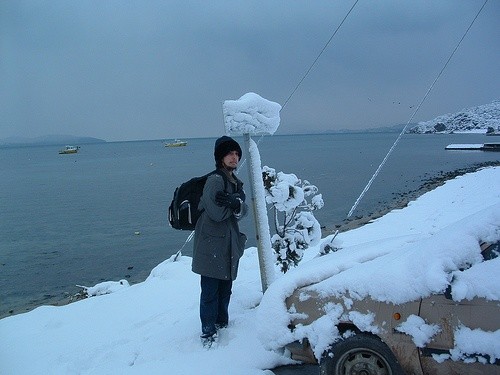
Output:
[167,141,187,147]
[59,144,79,154]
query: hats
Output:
[214,136,242,162]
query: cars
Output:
[286,167,500,375]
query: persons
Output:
[190,135,247,353]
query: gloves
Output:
[233,189,246,202]
[215,191,240,211]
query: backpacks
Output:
[168,170,228,231]
